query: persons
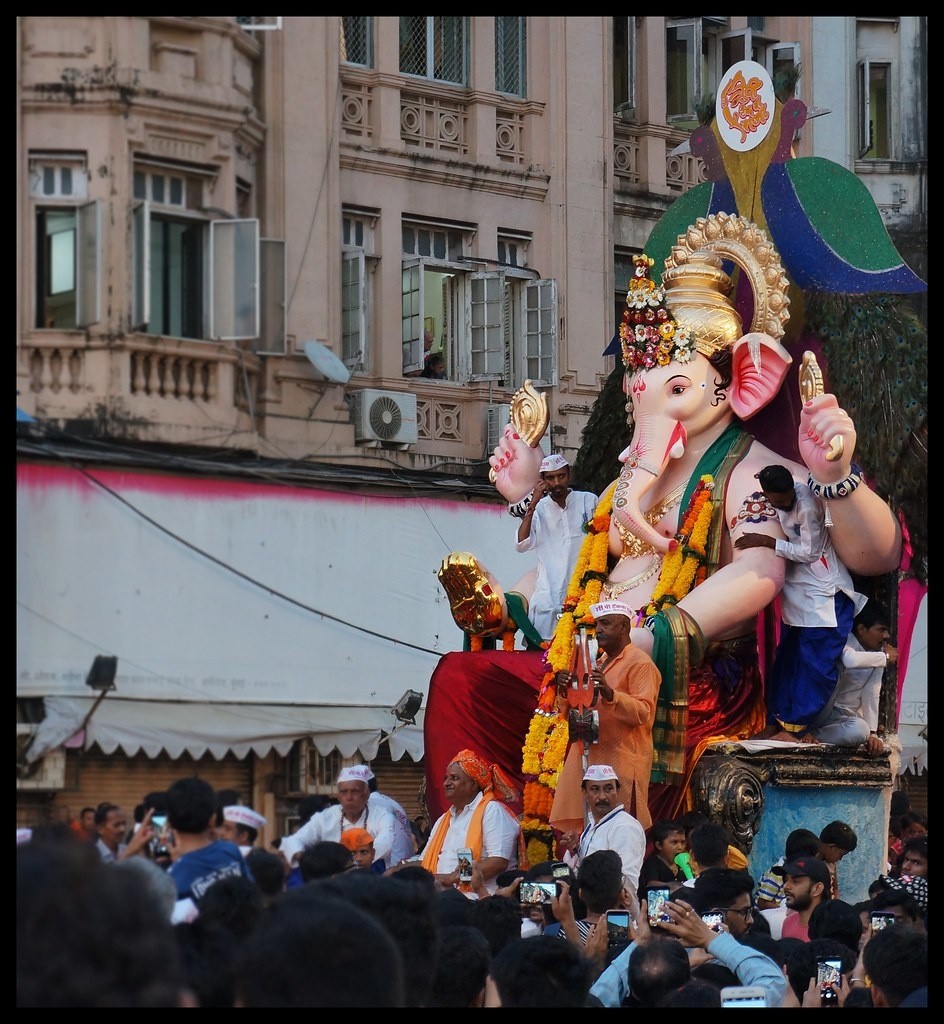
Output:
[733,465,867,743]
[801,603,898,760]
[425,210,903,834]
[564,764,645,922]
[353,765,411,869]
[16,777,927,1008]
[400,748,520,899]
[281,767,412,870]
[548,601,661,830]
[515,454,599,637]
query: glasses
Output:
[718,906,753,915]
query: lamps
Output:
[78,652,119,730]
[375,689,426,742]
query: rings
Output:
[690,909,694,913]
[686,912,689,918]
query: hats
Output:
[539,454,569,473]
[880,875,929,912]
[583,765,619,781]
[222,805,268,833]
[340,828,374,851]
[337,767,367,789]
[352,765,375,780]
[590,600,636,621]
[773,856,831,889]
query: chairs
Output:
[575,62,922,903]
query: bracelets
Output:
[509,489,548,517]
[886,654,889,659]
[480,894,491,901]
[808,462,863,500]
[644,616,655,636]
[851,979,865,982]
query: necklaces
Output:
[340,804,369,833]
[616,478,691,560]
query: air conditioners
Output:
[352,387,418,444]
[488,404,552,460]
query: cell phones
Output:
[457,848,473,881]
[551,862,570,878]
[815,956,844,1005]
[150,813,174,856]
[720,987,768,1008]
[871,911,894,938]
[645,885,670,930]
[517,881,561,905]
[700,911,724,955]
[605,910,630,949]
